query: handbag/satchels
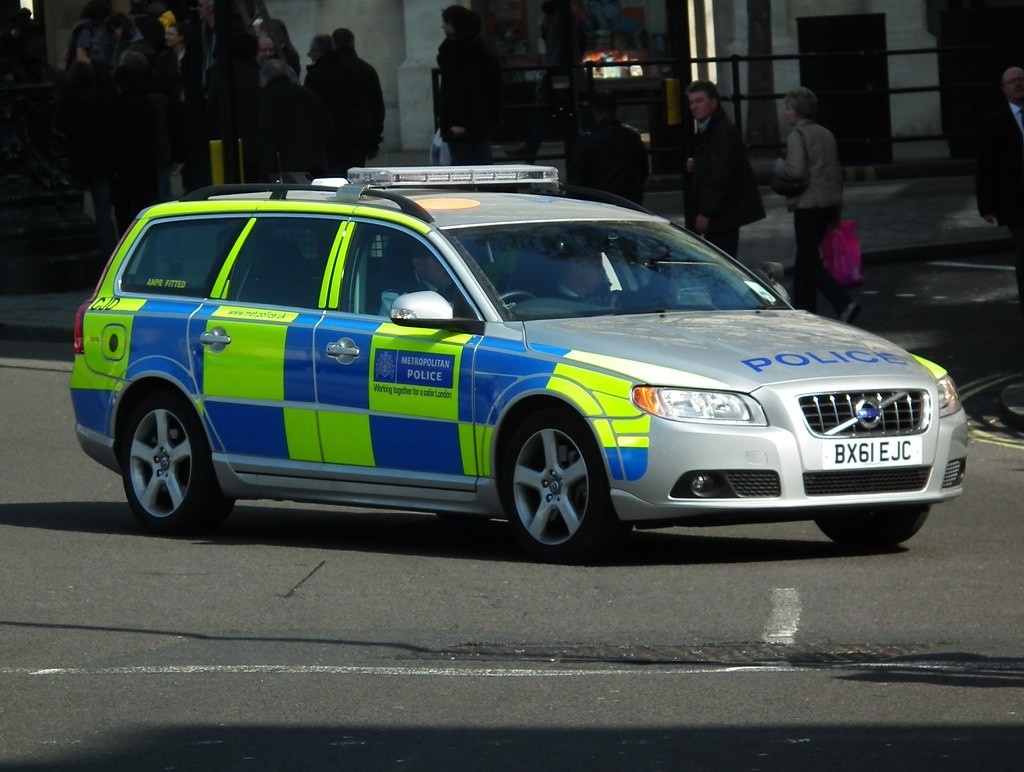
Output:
[770,129,810,198]
[820,220,866,290]
[169,169,184,199]
[430,127,451,165]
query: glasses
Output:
[107,26,121,36]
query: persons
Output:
[682,81,766,255]
[0,0,385,262]
[439,6,500,165]
[977,67,1024,316]
[776,87,868,323]
[563,92,649,210]
[377,242,484,317]
[554,242,669,307]
[505,0,586,164]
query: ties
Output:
[1019,109,1024,130]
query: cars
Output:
[69,164,969,562]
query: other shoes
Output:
[840,302,860,322]
